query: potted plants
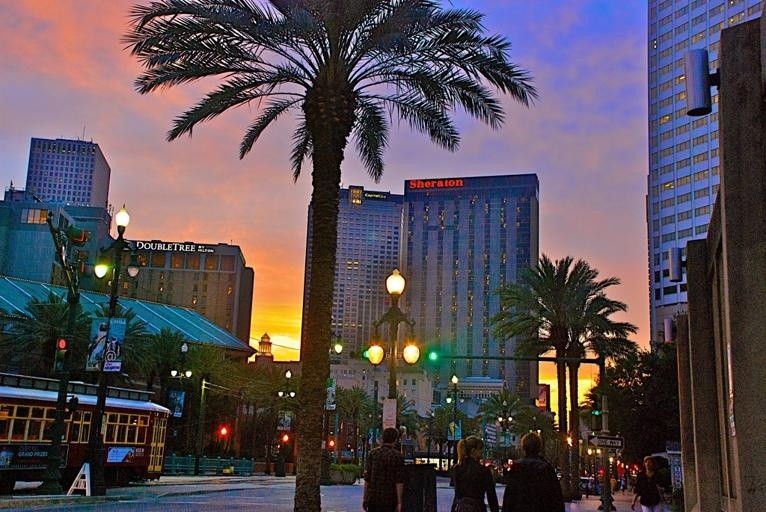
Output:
[328,463,363,484]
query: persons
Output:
[359,428,404,510]
[500,431,567,512]
[450,433,500,511]
[630,453,666,511]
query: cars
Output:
[479,458,625,498]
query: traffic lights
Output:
[350,350,369,360]
[420,351,440,361]
[68,223,93,248]
[52,337,71,376]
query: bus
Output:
[0,373,171,495]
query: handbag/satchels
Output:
[655,483,673,505]
[452,496,485,511]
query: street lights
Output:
[76,204,141,497]
[446,374,465,486]
[497,399,514,484]
[318,315,343,484]
[367,268,420,482]
[529,415,541,433]
[274,369,296,477]
[166,341,192,455]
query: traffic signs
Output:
[386,351,404,360]
[587,435,624,451]
